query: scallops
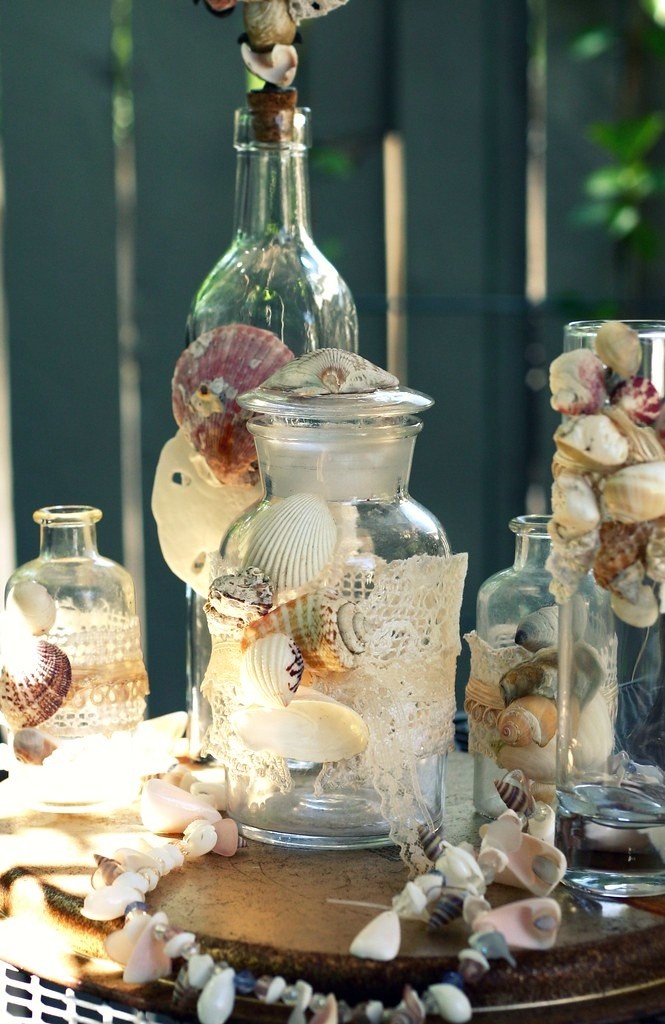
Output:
[1,321,664,1023]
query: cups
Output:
[555,318,665,899]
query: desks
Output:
[0,812,665,1024]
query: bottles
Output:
[201,344,464,855]
[187,88,359,361]
[472,514,616,827]
[2,503,152,745]
